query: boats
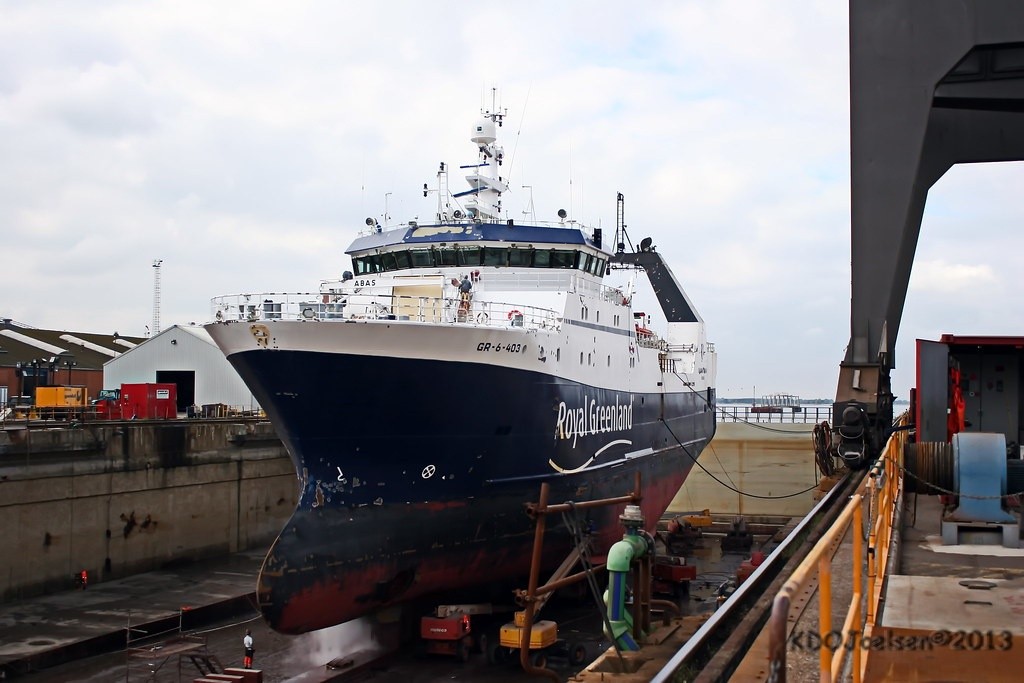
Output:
[202,83,718,636]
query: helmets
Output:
[246,629,251,634]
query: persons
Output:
[458,275,472,309]
[358,218,382,238]
